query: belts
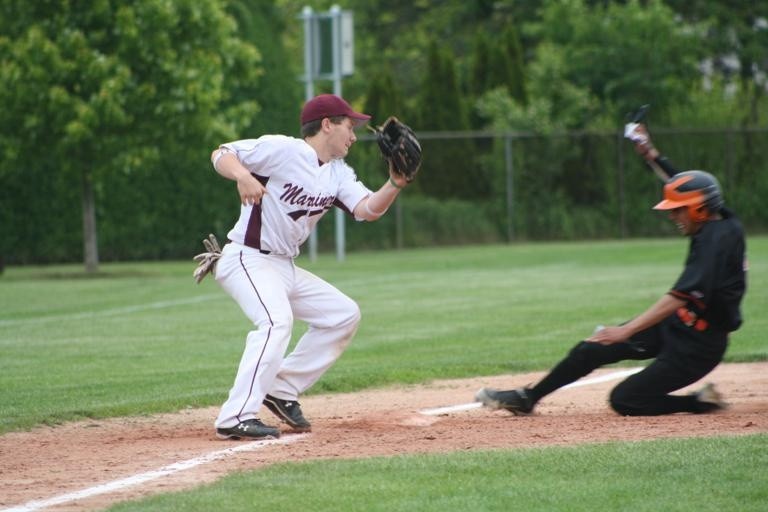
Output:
[675,303,709,332]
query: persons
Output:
[206,92,419,444]
[474,119,749,423]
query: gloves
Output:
[192,232,224,285]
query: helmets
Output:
[650,168,725,223]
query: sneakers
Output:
[261,393,312,433]
[473,384,537,417]
[215,418,283,442]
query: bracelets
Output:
[389,176,402,193]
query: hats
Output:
[300,93,374,126]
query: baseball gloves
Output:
[378,117,423,179]
